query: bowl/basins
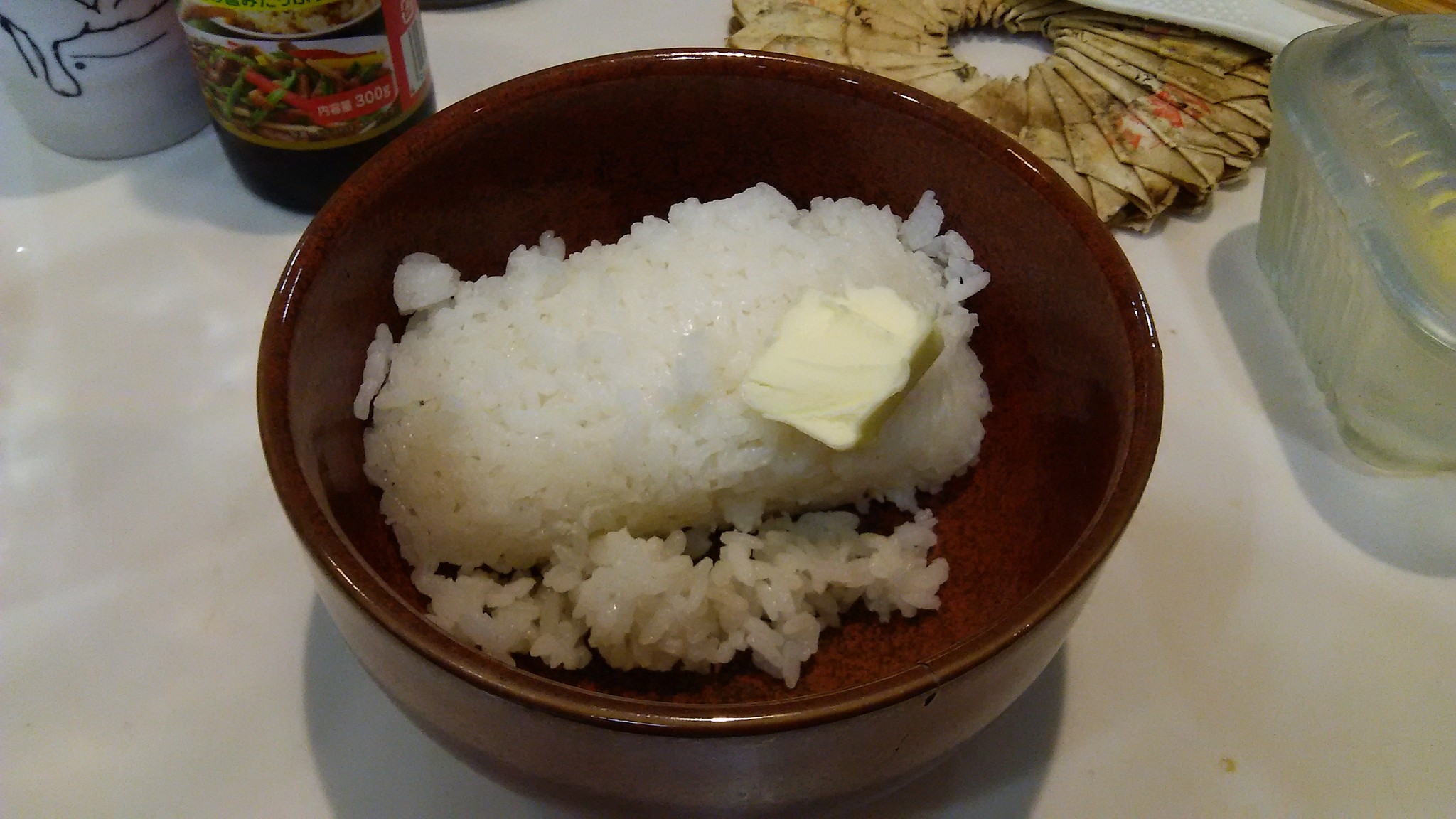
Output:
[259,44,1169,818]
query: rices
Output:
[365,170,1006,689]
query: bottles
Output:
[174,1,440,217]
[0,0,229,160]
